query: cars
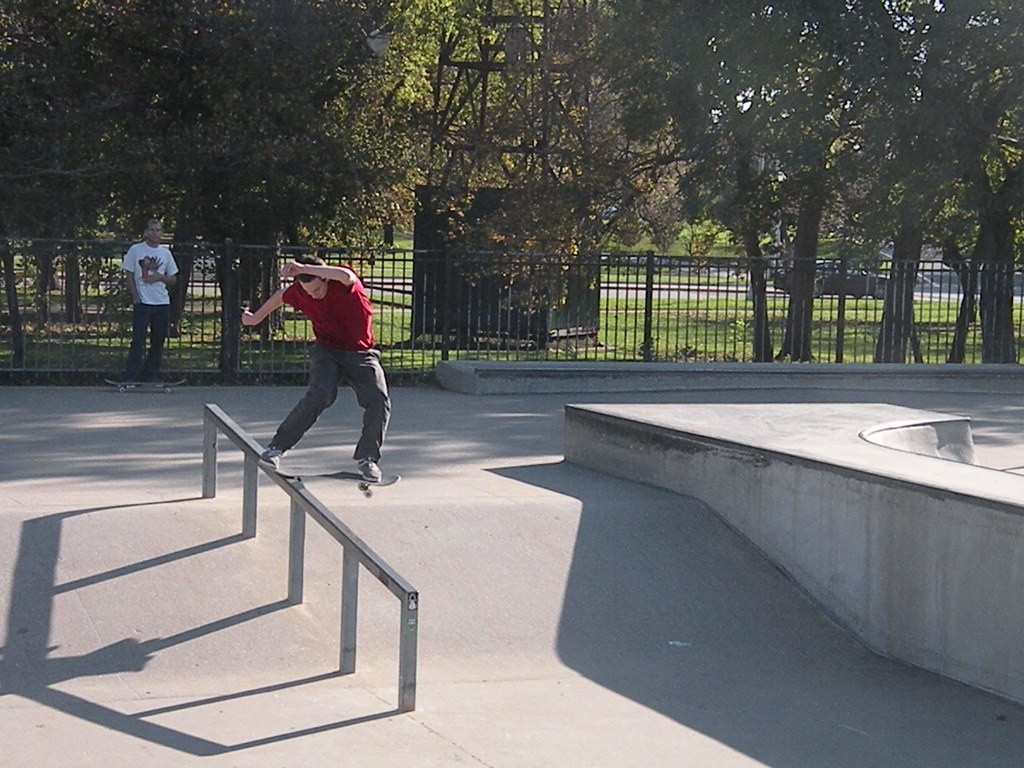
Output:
[160,244,240,282]
[776,259,890,299]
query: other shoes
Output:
[145,371,174,383]
[114,370,144,382]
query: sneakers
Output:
[356,458,381,481]
[263,447,282,470]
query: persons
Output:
[120,219,180,381]
[242,254,392,481]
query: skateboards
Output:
[267,467,402,499]
